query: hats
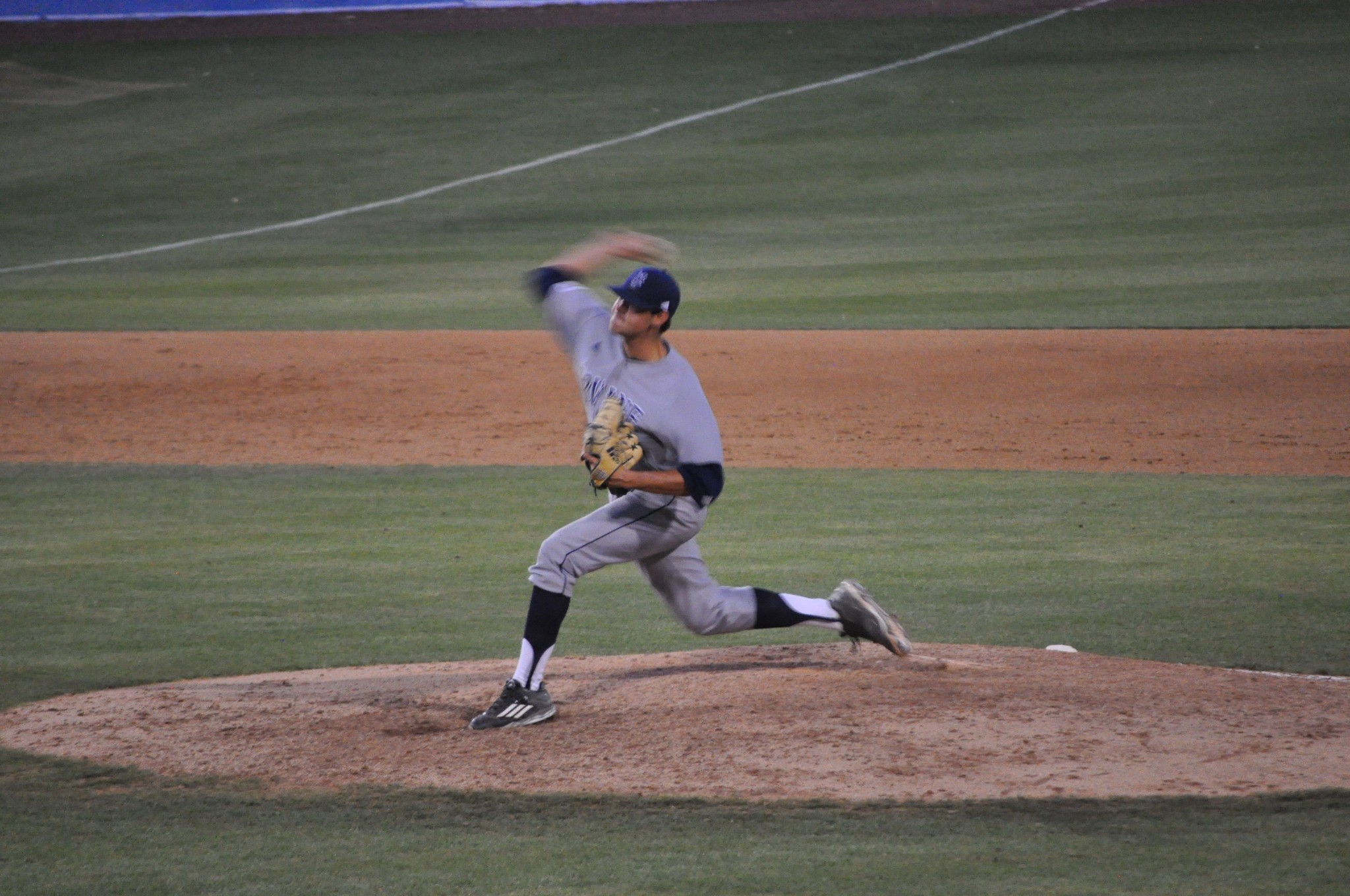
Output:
[608,267,680,316]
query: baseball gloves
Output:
[581,399,644,497]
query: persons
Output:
[462,230,911,732]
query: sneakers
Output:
[469,674,557,733]
[828,580,912,657]
